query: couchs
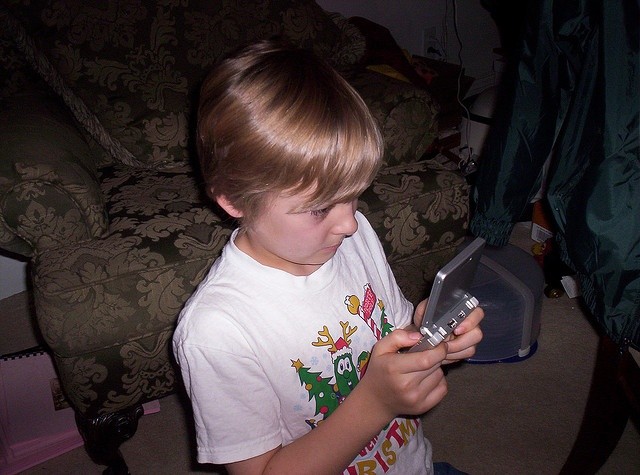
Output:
[0,0,473,475]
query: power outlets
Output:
[423,27,436,57]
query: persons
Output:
[172,37,484,475]
